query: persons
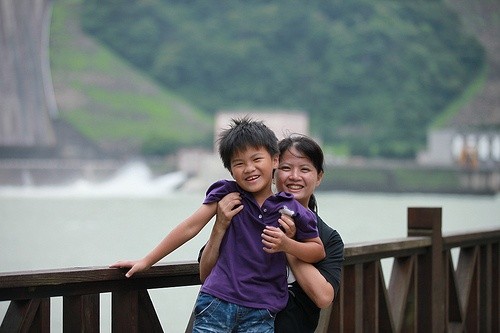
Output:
[197,132,345,333]
[108,114,326,332]
